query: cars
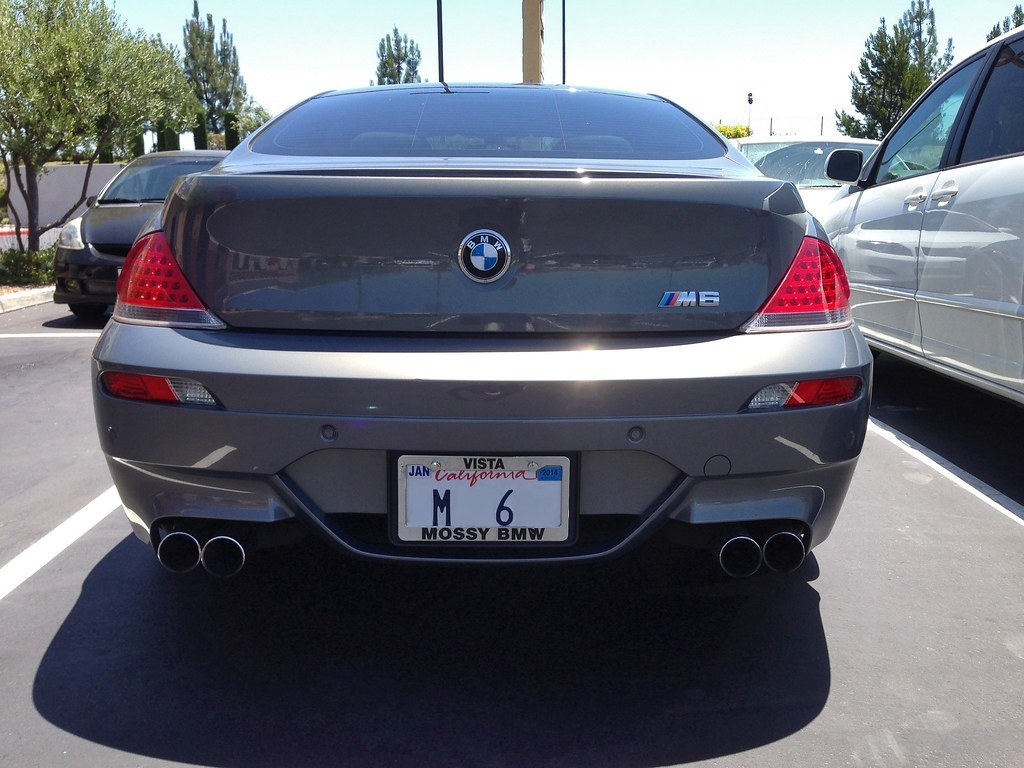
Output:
[728,134,880,221]
[51,150,234,320]
[819,23,1024,410]
[90,84,875,581]
